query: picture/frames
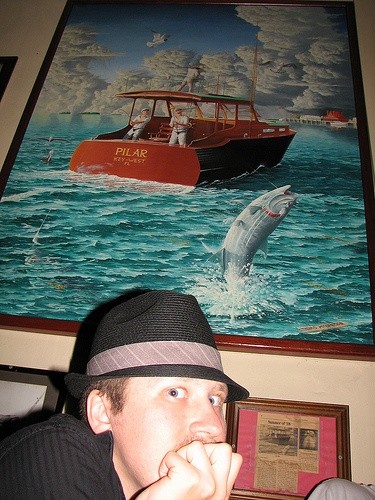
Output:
[1,1,375,361]
[222,395,350,500]
[0,366,67,421]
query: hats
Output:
[141,108,149,112]
[63,291,251,402]
[174,108,183,111]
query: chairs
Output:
[148,122,172,142]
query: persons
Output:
[0,291,249,500]
[169,107,189,146]
[123,108,151,141]
